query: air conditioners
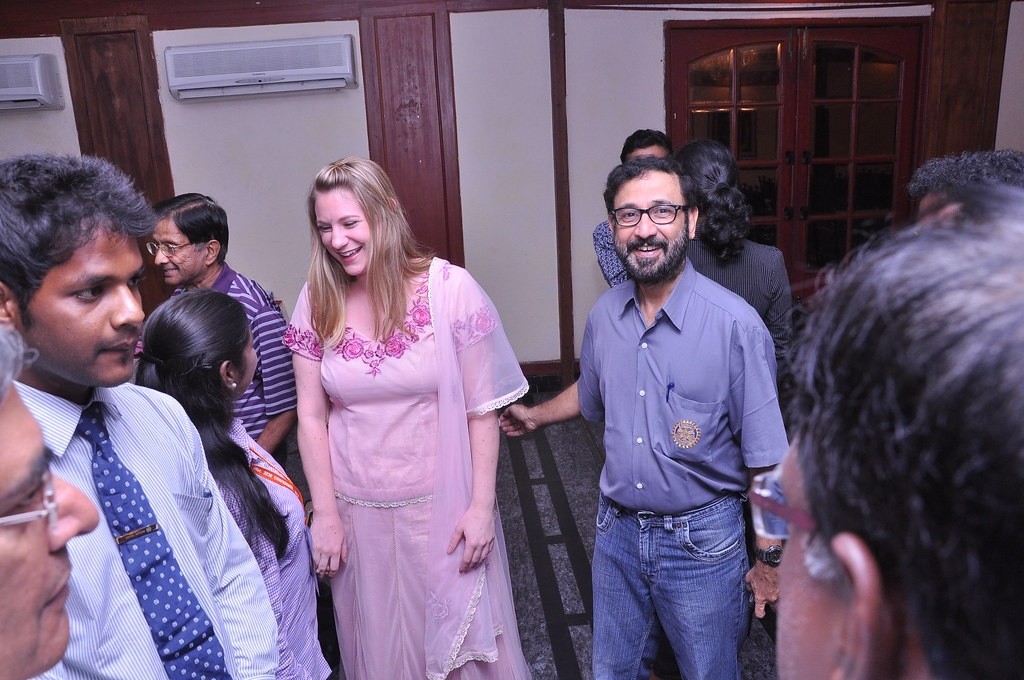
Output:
[1,53,67,118]
[162,35,361,105]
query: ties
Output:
[77,404,233,680]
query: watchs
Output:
[752,542,785,567]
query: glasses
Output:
[146,241,208,257]
[0,459,58,526]
[610,204,688,227]
[751,466,818,540]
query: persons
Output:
[906,149,1024,237]
[146,189,299,469]
[2,151,283,680]
[745,182,1023,680]
[497,158,791,680]
[1,327,101,680]
[277,155,536,680]
[666,138,797,439]
[590,129,677,286]
[135,289,332,678]
